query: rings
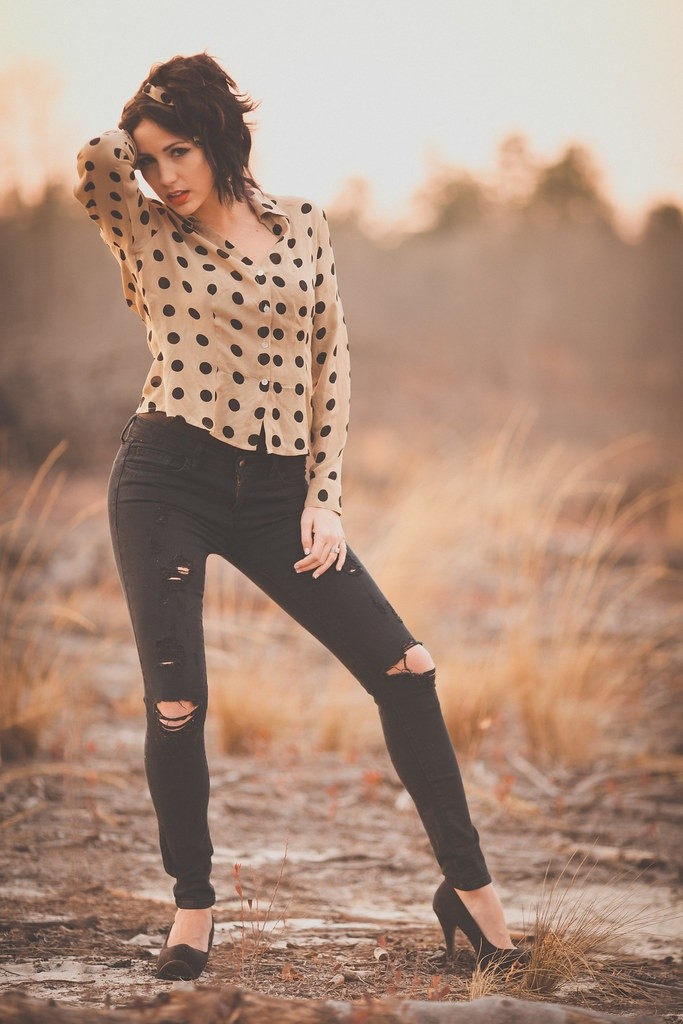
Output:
[330,549,339,553]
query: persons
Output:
[71,50,532,977]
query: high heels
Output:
[157,913,214,979]
[433,881,534,971]
